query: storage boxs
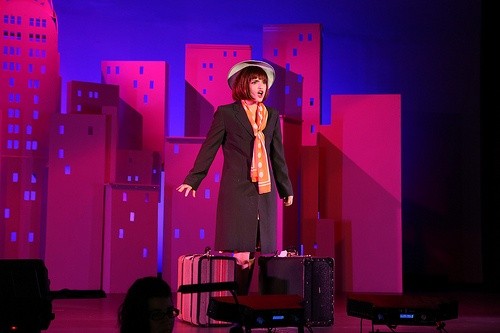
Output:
[176,254,236,326]
[257,256,335,327]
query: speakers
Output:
[0,258,55,333]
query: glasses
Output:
[147,305,179,320]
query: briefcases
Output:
[175,249,237,327]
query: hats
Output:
[227,59,276,90]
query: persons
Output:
[176,60,293,333]
[119,277,174,333]
